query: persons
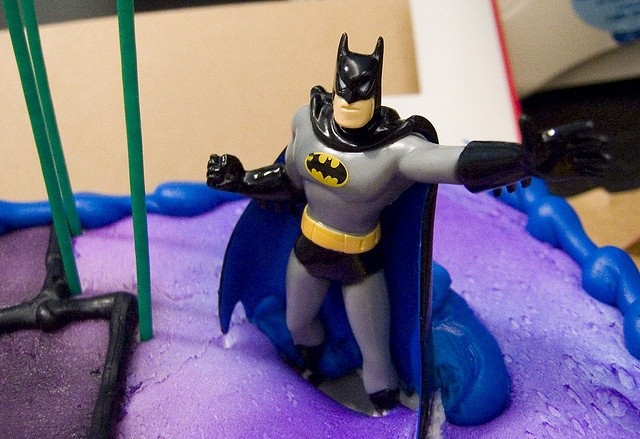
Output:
[206,33,612,439]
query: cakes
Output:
[0,177,639,439]
